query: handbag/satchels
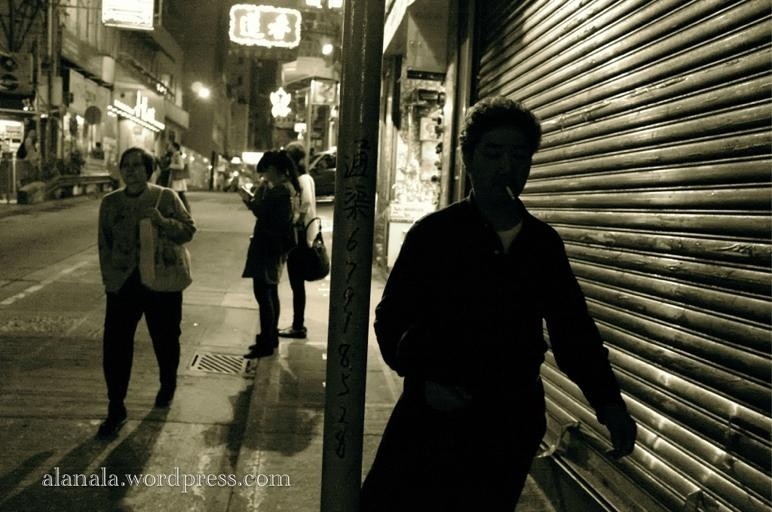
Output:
[140,217,193,291]
[293,234,329,281]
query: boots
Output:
[242,330,279,360]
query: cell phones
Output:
[241,186,254,197]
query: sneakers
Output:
[98,406,127,436]
[155,380,176,408]
[278,326,307,339]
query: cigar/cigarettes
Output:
[506,186,516,200]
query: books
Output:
[240,185,255,203]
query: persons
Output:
[360,93,640,511]
[91,141,104,160]
[275,140,319,339]
[167,141,192,213]
[155,142,173,187]
[97,145,198,441]
[240,146,303,359]
[23,128,36,160]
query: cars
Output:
[308,150,337,197]
